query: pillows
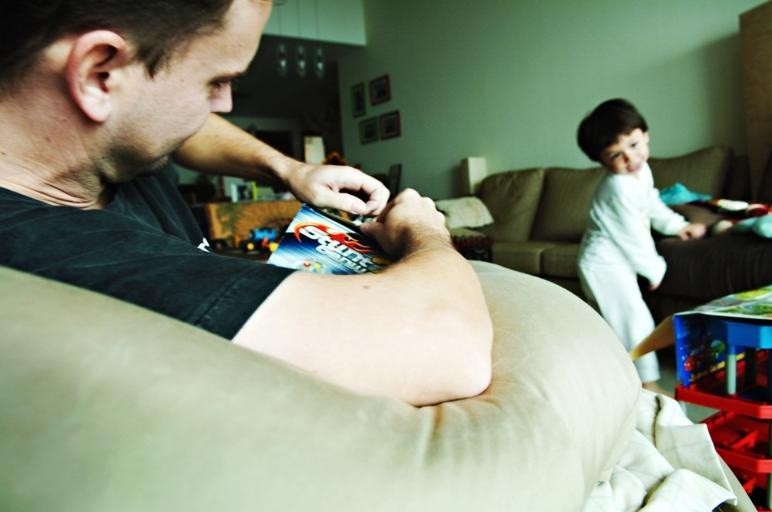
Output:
[480,145,733,241]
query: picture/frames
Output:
[350,74,402,145]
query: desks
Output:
[191,200,301,245]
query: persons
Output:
[0,0,495,409]
[577,98,707,401]
